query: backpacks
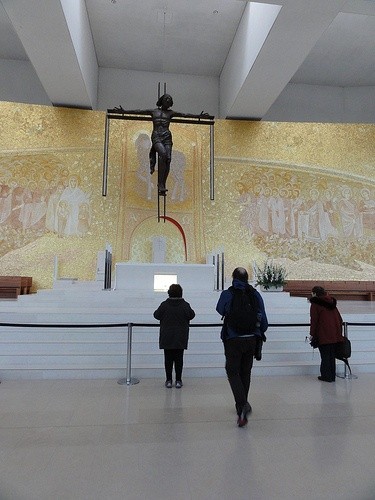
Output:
[228,285,258,335]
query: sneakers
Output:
[165,382,172,388]
[176,381,182,388]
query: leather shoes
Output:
[235,402,251,427]
[318,375,335,382]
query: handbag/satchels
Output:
[335,336,351,358]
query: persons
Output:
[153,282,196,389]
[307,286,343,382]
[215,267,269,425]
[113,92,210,196]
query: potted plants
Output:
[254,260,288,292]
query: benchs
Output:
[283,280,375,300]
[0,276,32,297]
